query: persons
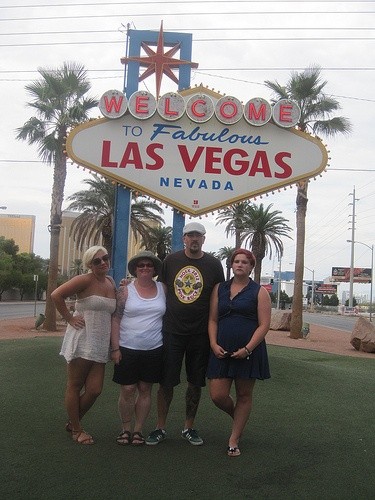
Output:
[120,222,225,446]
[111,251,168,446]
[206,249,270,456]
[51,245,117,446]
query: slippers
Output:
[227,445,240,456]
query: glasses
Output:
[137,262,155,268]
[92,254,110,265]
[187,232,203,239]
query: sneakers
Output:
[181,425,204,445]
[146,428,168,445]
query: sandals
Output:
[116,430,132,444]
[132,431,145,447]
[71,426,96,444]
[65,419,72,432]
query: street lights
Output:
[345,239,375,322]
[289,262,315,312]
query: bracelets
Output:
[111,349,120,352]
[244,347,250,355]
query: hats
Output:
[183,222,206,237]
[128,251,163,277]
